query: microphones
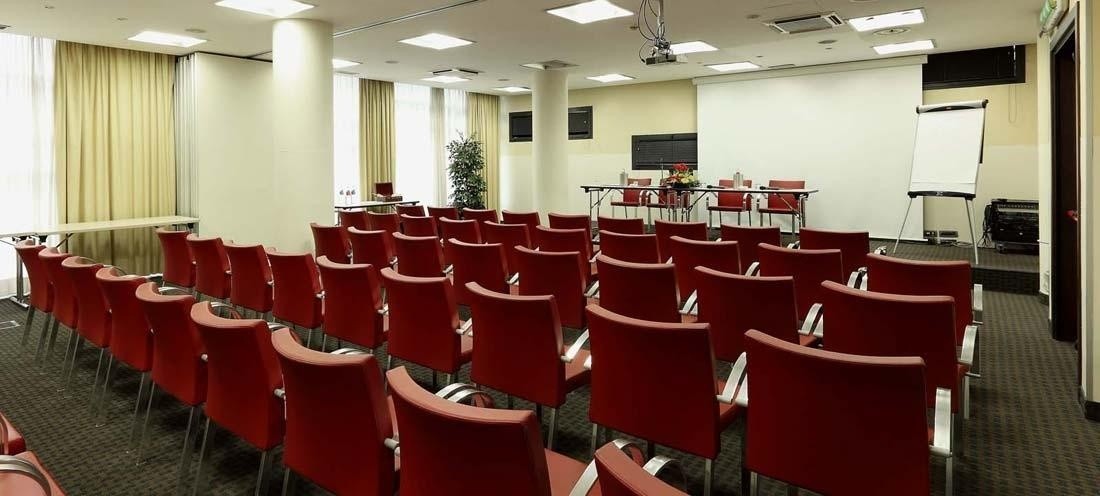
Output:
[760,186,779,190]
[707,185,724,189]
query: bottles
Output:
[620,169,629,187]
[733,171,744,189]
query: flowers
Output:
[665,163,698,187]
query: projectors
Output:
[646,55,688,67]
